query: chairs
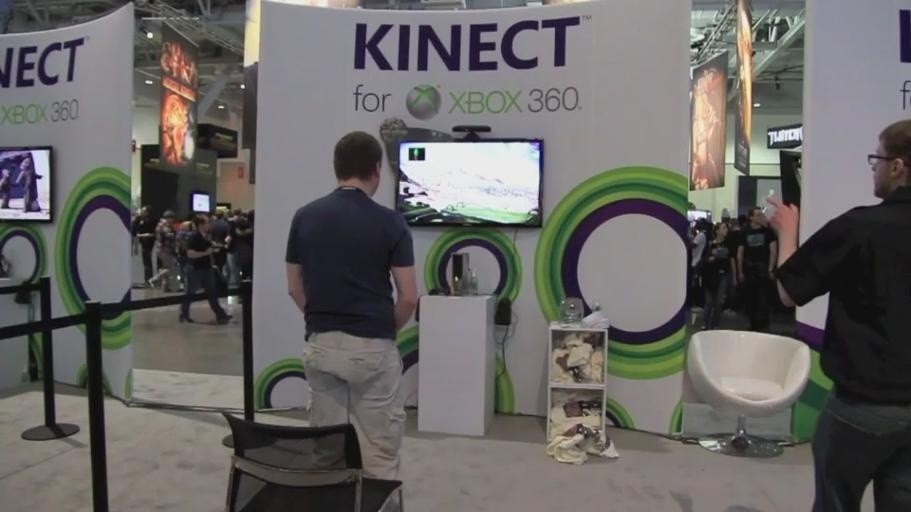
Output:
[687,329,812,458]
[221,408,405,512]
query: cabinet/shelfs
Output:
[545,321,612,450]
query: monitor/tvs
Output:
[0,146,53,223]
[779,150,802,211]
[687,209,711,227]
[395,137,543,228]
[192,192,211,213]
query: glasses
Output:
[867,154,895,167]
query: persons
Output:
[0,168,17,208]
[130,201,253,324]
[284,129,420,481]
[686,206,794,334]
[14,158,42,212]
[765,119,910,512]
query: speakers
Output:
[451,252,469,295]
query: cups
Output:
[464,271,479,296]
[558,297,584,329]
